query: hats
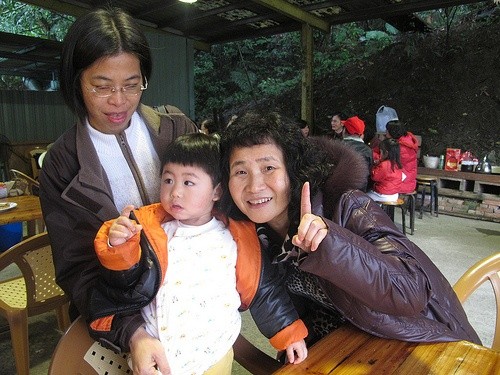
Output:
[341,117,365,135]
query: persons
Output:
[37,6,200,375]
[384,119,418,193]
[200,117,221,139]
[71,133,310,375]
[296,118,311,137]
[218,112,486,364]
[365,139,403,204]
[339,116,373,168]
[325,113,345,140]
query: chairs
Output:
[9,169,40,233]
[30,148,46,178]
[453,253,500,352]
[0,232,71,375]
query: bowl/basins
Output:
[461,165,474,172]
[421,154,439,169]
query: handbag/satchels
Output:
[376,105,399,133]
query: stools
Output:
[401,191,417,236]
[416,175,438,216]
[416,182,440,217]
[379,199,407,235]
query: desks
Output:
[271,324,500,375]
[0,195,44,235]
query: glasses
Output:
[79,77,150,96]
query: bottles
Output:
[437,155,445,170]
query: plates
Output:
[0,202,17,212]
